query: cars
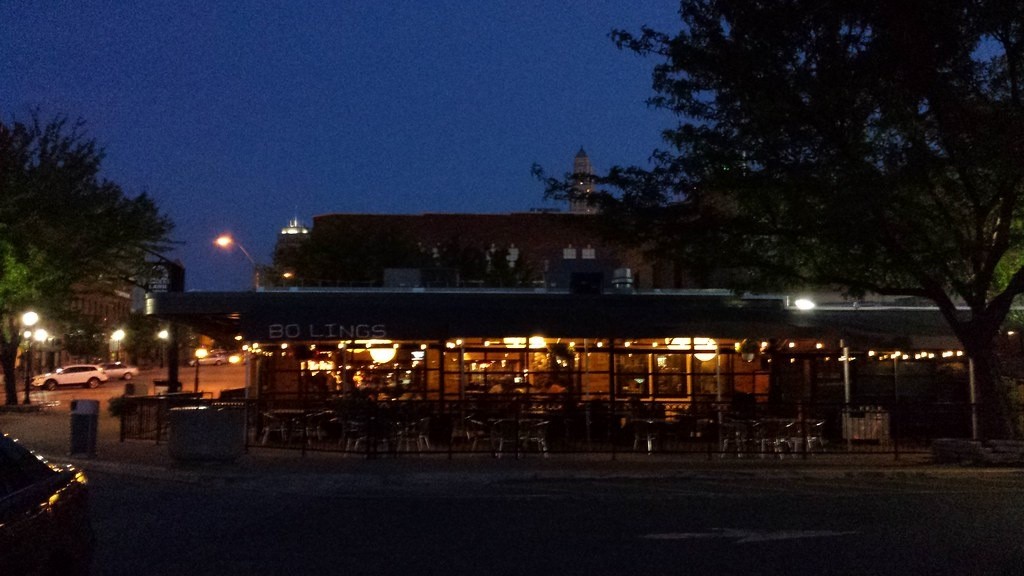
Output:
[98,362,141,380]
[1,428,97,576]
[186,353,227,367]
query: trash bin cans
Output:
[167,402,245,462]
[70,398,99,455]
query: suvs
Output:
[30,364,110,392]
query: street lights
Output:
[157,330,169,369]
[216,235,258,400]
[111,330,126,362]
[22,310,51,404]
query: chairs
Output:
[724,418,827,460]
[254,403,717,462]
